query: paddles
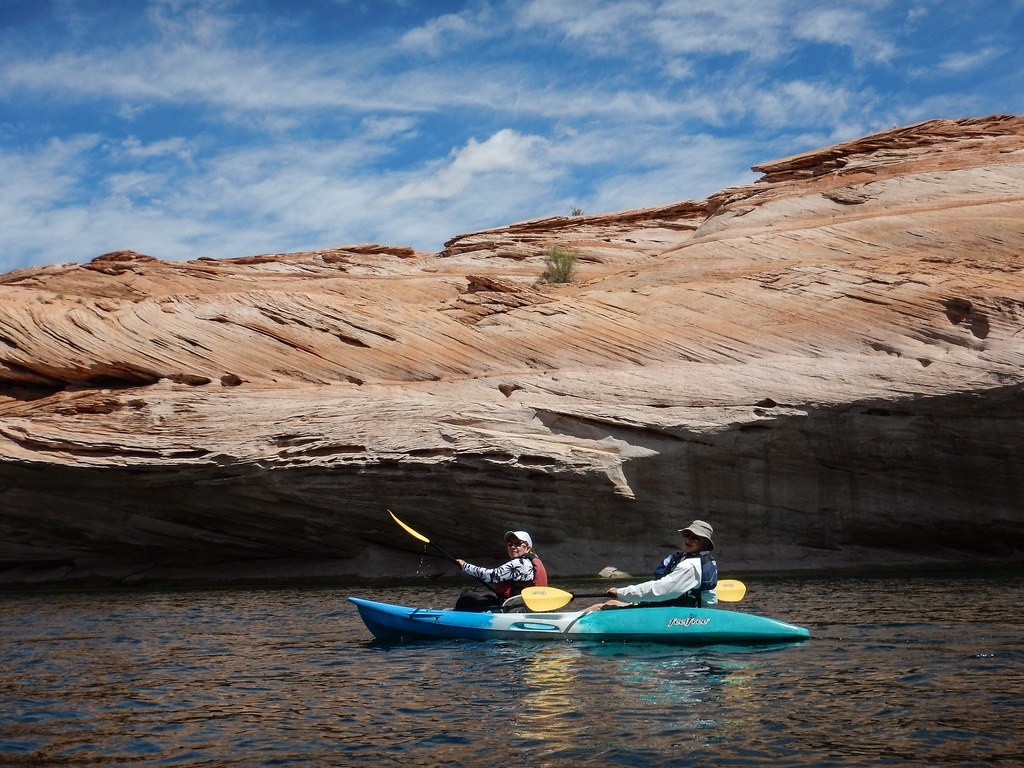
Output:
[387,509,496,594]
[521,578,748,613]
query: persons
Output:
[579,520,718,613]
[453,530,547,613]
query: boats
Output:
[344,596,810,645]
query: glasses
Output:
[507,540,528,547]
[684,531,702,545]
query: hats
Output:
[678,519,715,551]
[504,531,532,549]
